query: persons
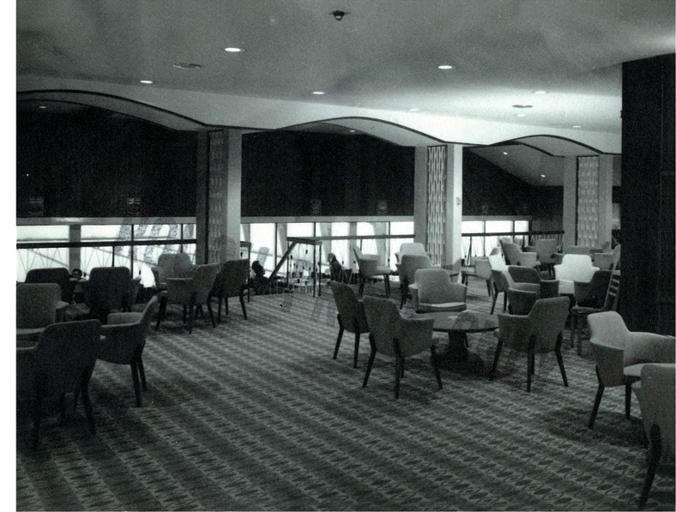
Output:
[69,269,86,304]
